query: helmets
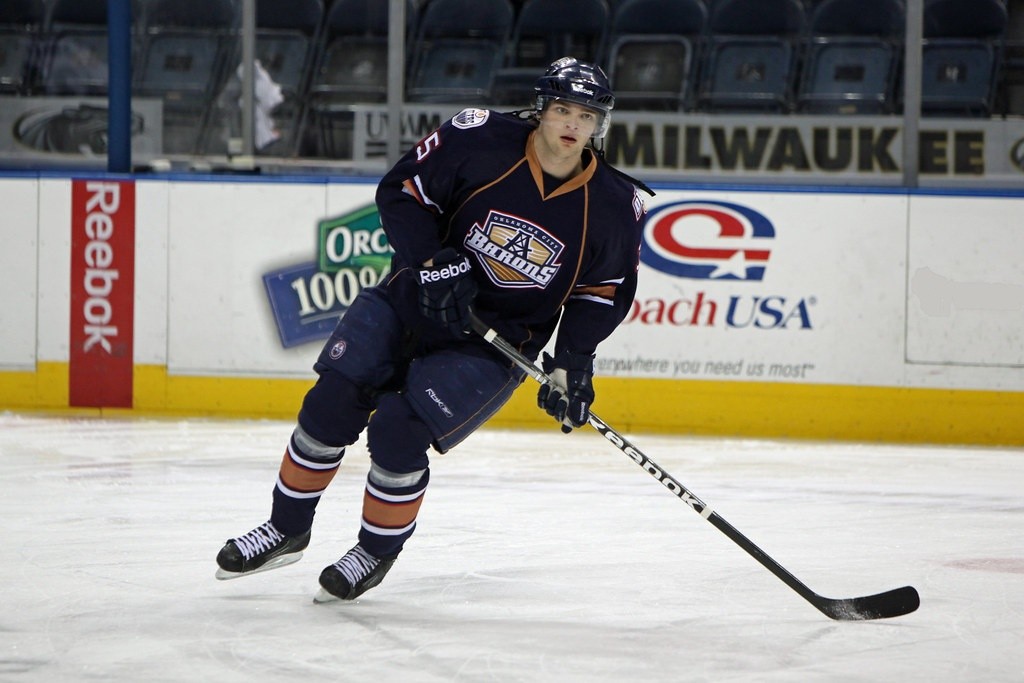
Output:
[534,56,616,111]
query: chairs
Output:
[0,0,1010,160]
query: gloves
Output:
[419,248,476,340]
[537,347,595,434]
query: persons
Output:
[214,54,644,604]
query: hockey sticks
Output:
[460,306,922,623]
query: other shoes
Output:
[215,520,311,580]
[312,541,404,605]
